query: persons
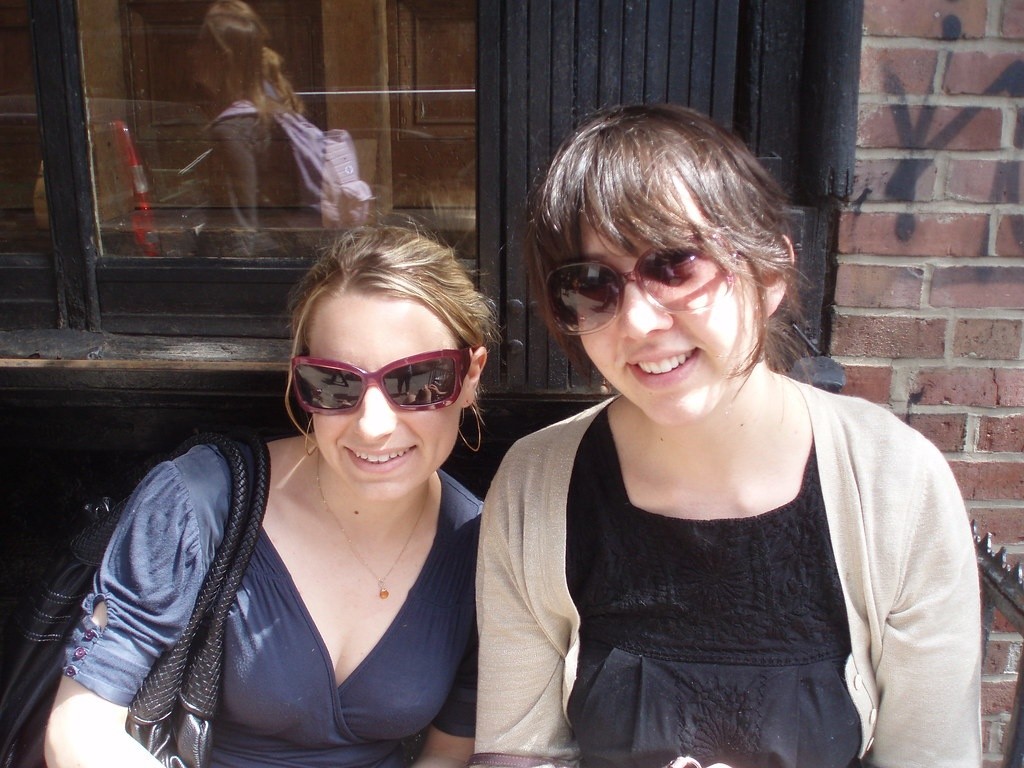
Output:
[185,0,308,257]
[44,223,492,768]
[475,104,981,768]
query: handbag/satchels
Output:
[0,430,271,768]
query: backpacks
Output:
[203,98,378,250]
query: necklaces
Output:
[316,453,429,599]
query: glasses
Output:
[291,345,476,415]
[543,244,746,336]
